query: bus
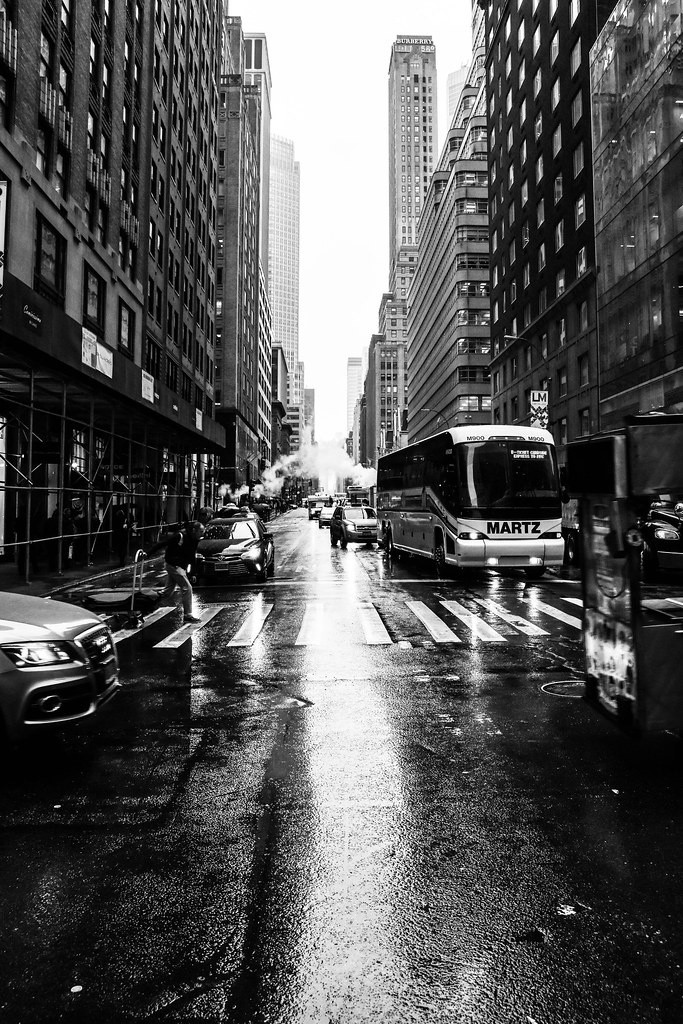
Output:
[376,425,565,581]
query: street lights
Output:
[503,334,550,371]
[422,407,450,429]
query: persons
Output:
[159,522,204,623]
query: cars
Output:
[0,590,122,752]
[191,517,275,583]
[328,506,379,550]
[304,486,370,529]
[566,408,683,743]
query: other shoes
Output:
[161,600,175,606]
[183,614,202,623]
